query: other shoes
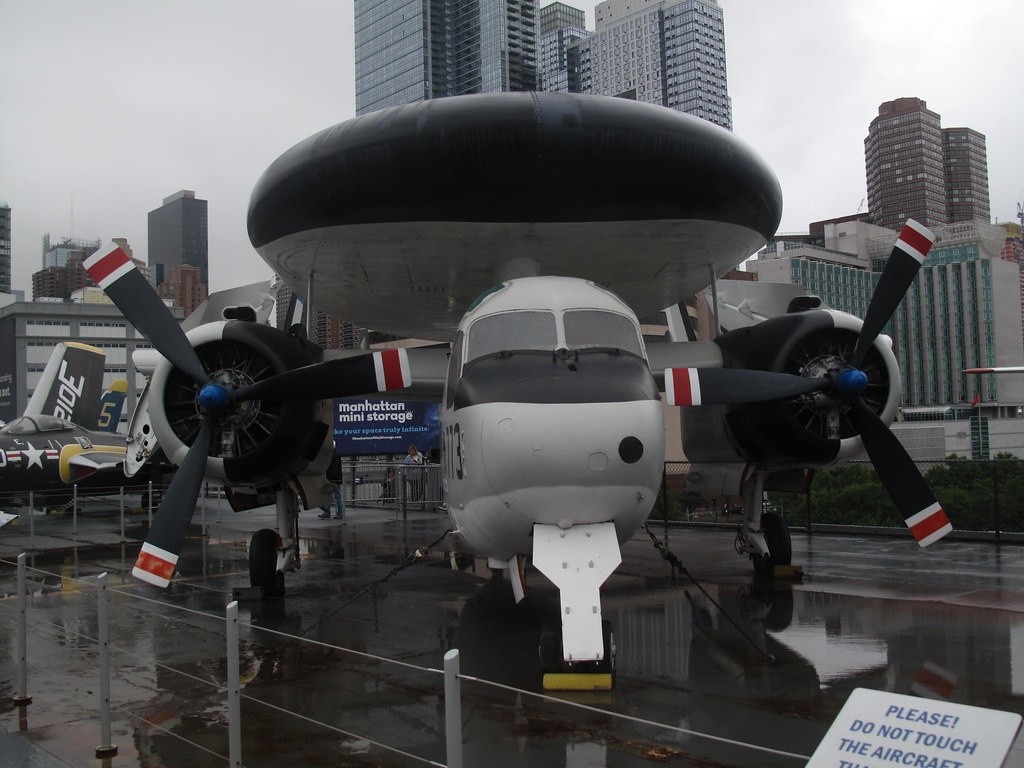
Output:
[318,512,331,518]
[333,514,343,519]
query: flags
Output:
[971,395,979,406]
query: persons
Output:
[318,440,343,519]
[404,446,428,502]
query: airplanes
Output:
[81,89,957,691]
[0,342,177,506]
